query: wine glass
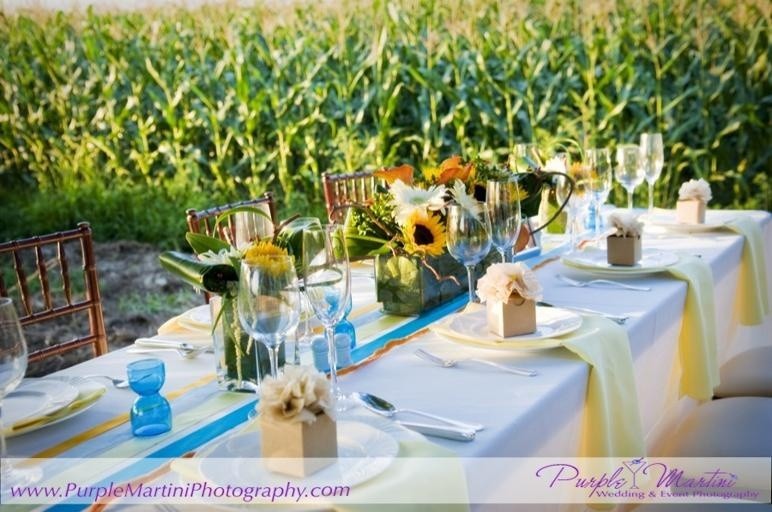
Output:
[444,200,493,303]
[0,298,45,495]
[301,223,352,411]
[485,177,522,263]
[236,253,302,377]
[583,132,664,236]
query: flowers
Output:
[157,139,601,379]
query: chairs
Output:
[0,222,109,364]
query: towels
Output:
[426,214,771,510]
[170,411,470,512]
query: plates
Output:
[190,420,400,497]
[1,378,80,430]
[447,305,583,341]
[561,245,679,279]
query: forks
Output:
[415,349,537,377]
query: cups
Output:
[512,142,541,175]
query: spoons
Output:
[84,372,152,388]
[556,273,652,291]
[353,392,485,431]
